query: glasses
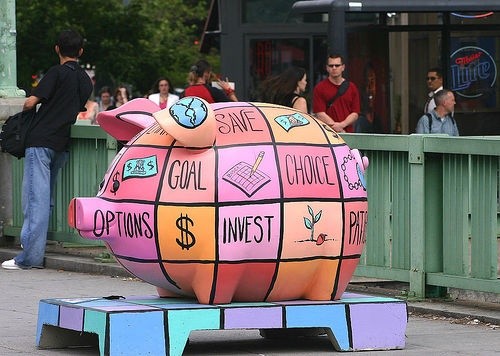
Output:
[327,64,342,68]
[426,77,440,81]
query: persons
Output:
[416,89,459,136]
[312,54,360,133]
[77,85,132,125]
[270,67,308,113]
[423,68,444,114]
[1,31,93,270]
[179,61,238,104]
[148,77,180,110]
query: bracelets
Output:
[226,88,234,95]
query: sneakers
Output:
[1,259,21,269]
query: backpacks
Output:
[0,105,35,159]
